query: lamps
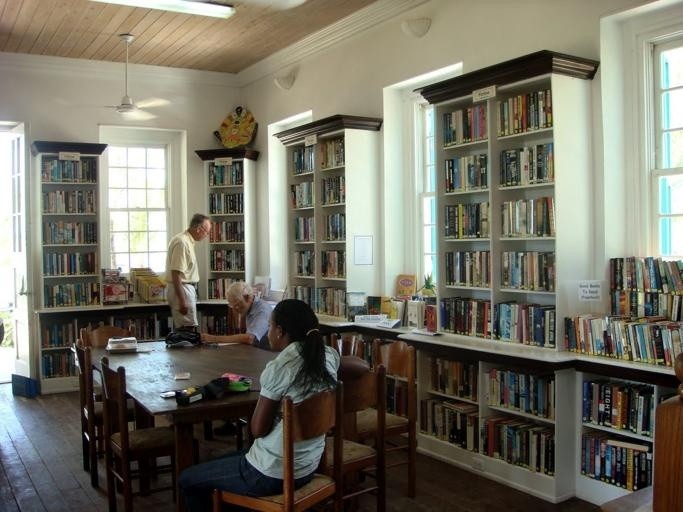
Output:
[89,2,237,21]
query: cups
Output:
[86,252,95,274]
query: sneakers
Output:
[213,422,245,437]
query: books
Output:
[358,336,374,369]
[481,414,554,476]
[41,160,99,378]
[423,353,478,401]
[582,376,654,438]
[492,300,555,349]
[445,250,491,288]
[564,312,681,368]
[290,139,346,318]
[440,296,491,339]
[501,251,555,292]
[419,398,479,453]
[500,143,554,187]
[608,257,681,322]
[366,295,438,334]
[486,367,555,422]
[445,201,489,239]
[103,265,166,305]
[210,161,244,299]
[500,197,556,238]
[581,435,653,491]
[496,89,553,137]
[442,104,488,146]
[445,154,488,192]
[103,311,228,341]
[385,377,411,416]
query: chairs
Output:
[71,325,417,511]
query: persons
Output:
[201,281,273,350]
[179,299,342,512]
[167,214,212,328]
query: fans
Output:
[64,33,170,122]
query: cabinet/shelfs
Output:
[30,141,108,308]
[194,148,259,300]
[397,333,575,505]
[414,49,600,352]
[34,300,240,398]
[575,358,681,506]
[272,114,383,318]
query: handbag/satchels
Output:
[165,331,201,346]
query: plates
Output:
[105,347,138,352]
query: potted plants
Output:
[416,273,438,297]
